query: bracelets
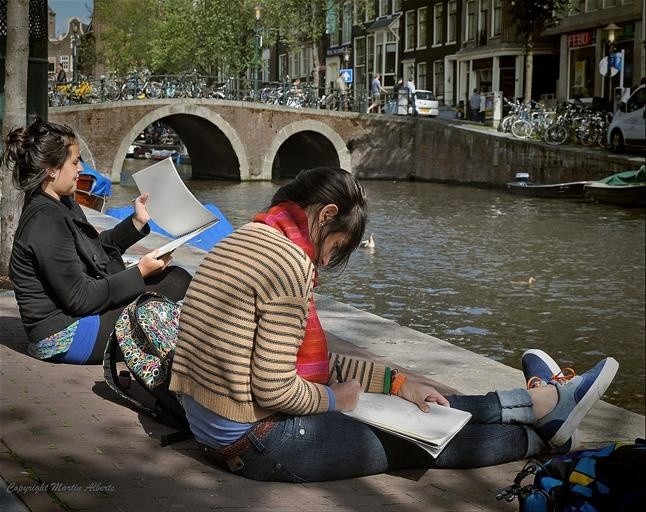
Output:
[390,373,408,396]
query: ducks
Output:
[511,276,537,287]
[359,231,376,249]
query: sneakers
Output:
[538,357,618,447]
[522,348,578,454]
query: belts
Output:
[199,414,275,462]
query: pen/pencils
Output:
[336,365,342,383]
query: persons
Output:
[289,78,304,97]
[167,163,620,483]
[406,78,418,115]
[365,72,388,113]
[334,70,350,112]
[392,75,404,100]
[1,114,193,364]
[570,96,586,114]
[470,88,480,122]
[56,62,67,90]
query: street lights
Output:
[604,22,621,100]
[251,4,262,101]
[70,21,80,82]
[342,47,351,68]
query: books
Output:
[335,389,473,460]
[124,154,221,270]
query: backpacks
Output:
[102,292,194,446]
[498,437,646,512]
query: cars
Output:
[607,84,646,151]
[381,90,439,116]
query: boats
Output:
[506,180,590,199]
[584,169,646,202]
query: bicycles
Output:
[245,75,355,110]
[498,96,613,148]
[47,69,232,107]
